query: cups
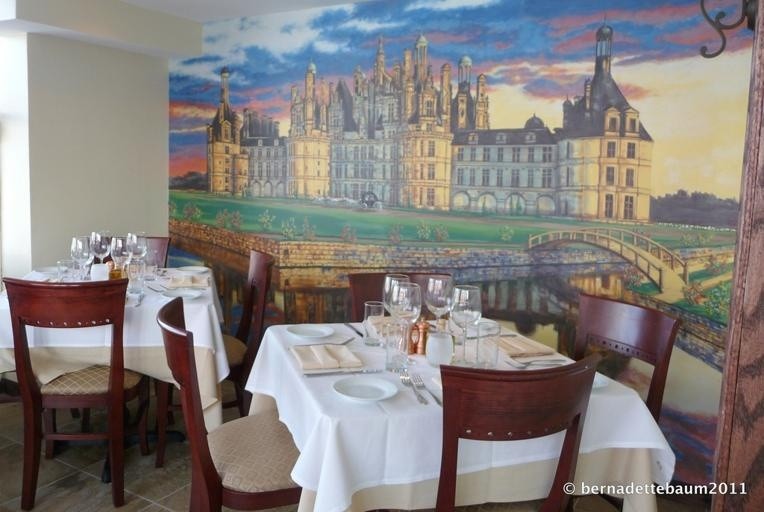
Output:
[362,301,383,346]
[144,255,157,281]
[425,332,452,367]
[56,260,76,284]
[127,259,145,295]
[476,321,500,369]
[383,323,408,373]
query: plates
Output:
[332,375,397,403]
[160,288,201,300]
[177,265,208,274]
[286,322,334,340]
[467,316,498,338]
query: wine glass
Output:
[71,230,147,283]
[423,274,453,329]
[448,284,482,366]
[389,282,420,366]
[382,274,410,349]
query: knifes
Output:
[344,322,363,338]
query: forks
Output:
[504,356,567,369]
[399,369,428,405]
[414,375,442,406]
